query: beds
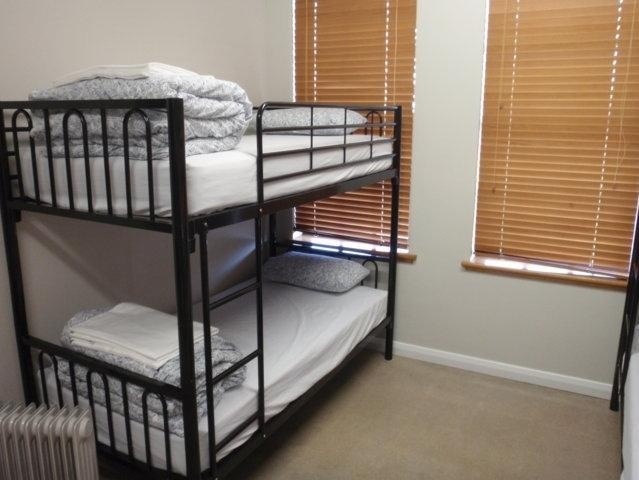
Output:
[607,208,639,479]
[0,100,401,479]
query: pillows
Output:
[262,250,368,294]
[249,107,368,135]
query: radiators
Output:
[1,400,96,480]
